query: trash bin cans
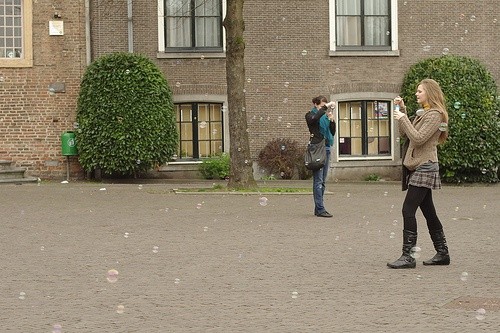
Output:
[62,130,78,156]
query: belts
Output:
[326,146,330,150]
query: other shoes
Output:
[314,210,317,216]
[317,210,333,217]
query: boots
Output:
[423,229,450,266]
[387,230,417,269]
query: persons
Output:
[305,95,337,218]
[387,78,451,269]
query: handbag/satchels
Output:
[304,142,326,170]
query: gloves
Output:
[325,101,336,109]
